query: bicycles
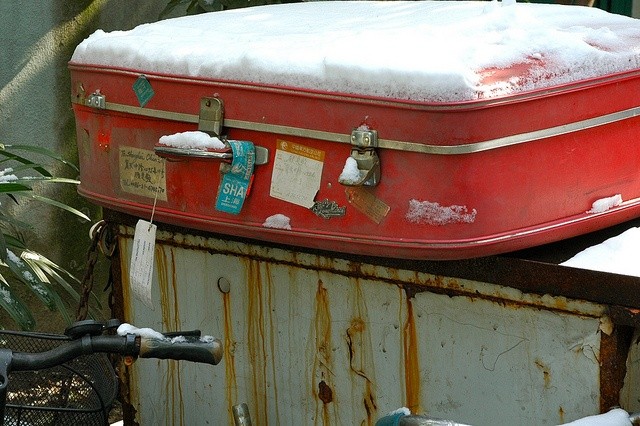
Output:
[0,319,471,426]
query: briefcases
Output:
[67,0,636,261]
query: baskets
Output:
[0,330,118,426]
